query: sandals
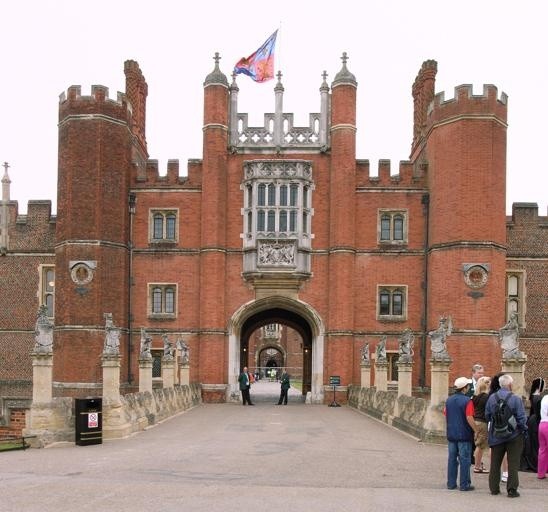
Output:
[473,465,489,473]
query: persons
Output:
[254,368,280,381]
[500,307,526,362]
[442,361,548,497]
[33,304,52,348]
[238,365,255,406]
[102,311,122,357]
[395,330,415,363]
[138,329,151,356]
[259,241,294,265]
[426,313,453,364]
[177,334,189,357]
[275,367,290,406]
[360,340,370,363]
[160,331,175,358]
[375,335,389,364]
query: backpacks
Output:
[491,392,518,440]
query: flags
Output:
[233,28,277,83]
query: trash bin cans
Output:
[74,396,103,446]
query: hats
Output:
[454,377,472,390]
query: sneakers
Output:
[501,476,508,482]
[491,490,499,495]
[507,488,520,497]
[460,486,475,491]
[447,484,457,489]
